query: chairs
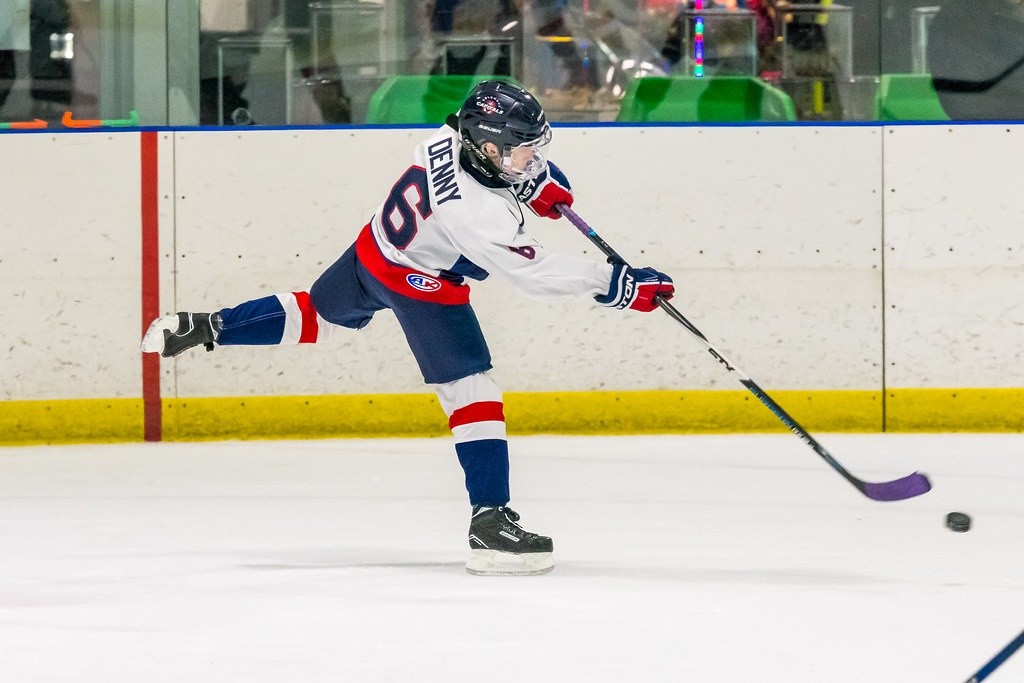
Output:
[615,73,797,124]
[871,74,951,122]
[364,76,530,125]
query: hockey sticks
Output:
[930,55,1024,94]
[964,629,1024,683]
[555,201,935,504]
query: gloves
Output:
[593,263,675,312]
[515,155,574,219]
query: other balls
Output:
[946,512,971,533]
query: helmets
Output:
[458,79,552,183]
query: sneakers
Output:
[140,310,222,357]
[465,503,555,576]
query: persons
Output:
[0,1,793,125]
[139,78,675,576]
